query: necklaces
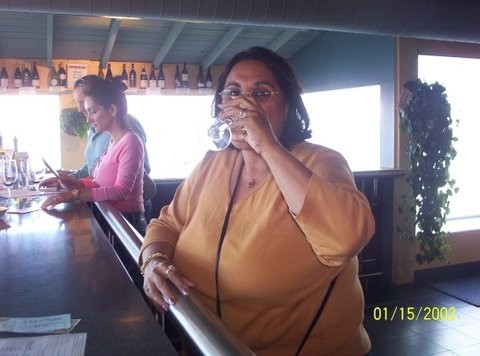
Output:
[109,128,134,146]
[244,165,270,189]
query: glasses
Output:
[74,79,90,88]
[217,87,284,104]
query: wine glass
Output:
[200,102,248,151]
[0,159,19,206]
[25,156,46,208]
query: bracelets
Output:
[140,252,172,275]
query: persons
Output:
[139,46,374,355]
[42,78,147,236]
[38,74,153,223]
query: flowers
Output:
[59,105,94,139]
[397,79,462,266]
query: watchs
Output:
[72,189,80,202]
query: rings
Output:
[166,264,177,275]
[147,285,151,293]
[240,108,247,119]
[241,134,247,142]
